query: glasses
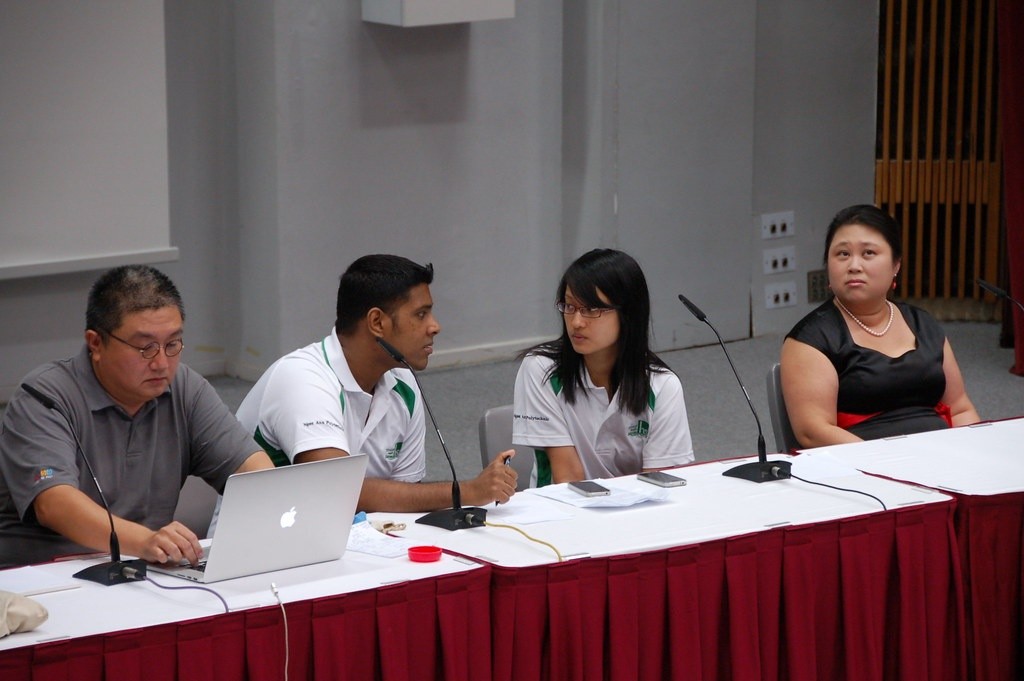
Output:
[96,322,183,359]
[556,300,618,319]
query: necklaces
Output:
[835,294,893,337]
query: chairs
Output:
[480,400,536,496]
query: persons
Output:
[205,251,518,541]
[503,247,695,487]
[0,263,283,572]
[779,204,981,453]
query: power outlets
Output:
[760,210,799,307]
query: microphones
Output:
[977,278,1024,313]
[21,382,148,586]
[375,336,487,531]
[678,293,792,483]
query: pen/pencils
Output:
[494,455,511,507]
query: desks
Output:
[1,416,1024,681]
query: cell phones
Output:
[569,481,610,498]
[637,471,686,488]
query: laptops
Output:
[146,453,371,583]
